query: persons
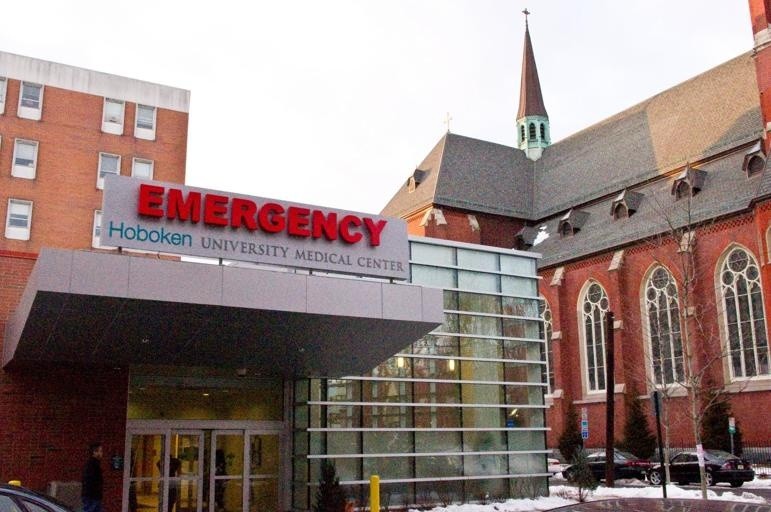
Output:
[209,449,228,512]
[81,442,103,512]
[156,452,181,512]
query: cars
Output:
[506,408,525,428]
[547,458,564,482]
[561,448,654,488]
[646,446,757,487]
[0,483,76,512]
